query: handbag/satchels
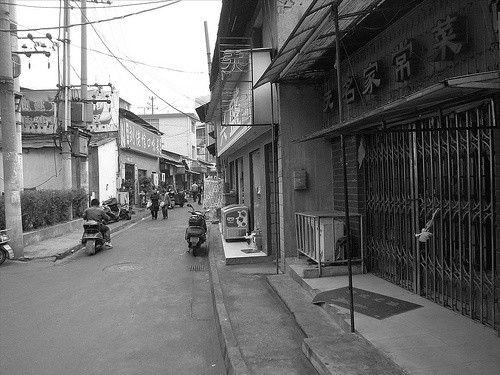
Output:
[147,201,153,208]
[160,202,165,207]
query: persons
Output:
[148,181,202,219]
[84,200,113,248]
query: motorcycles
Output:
[82,219,107,257]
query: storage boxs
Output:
[189,215,205,226]
[82,220,101,232]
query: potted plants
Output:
[139,176,152,207]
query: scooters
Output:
[0,229,14,265]
[184,203,211,256]
[101,194,132,225]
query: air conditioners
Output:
[304,218,344,262]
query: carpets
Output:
[312,285,425,323]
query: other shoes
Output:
[152,217,154,220]
[164,216,168,219]
[155,218,157,220]
[105,242,112,247]
